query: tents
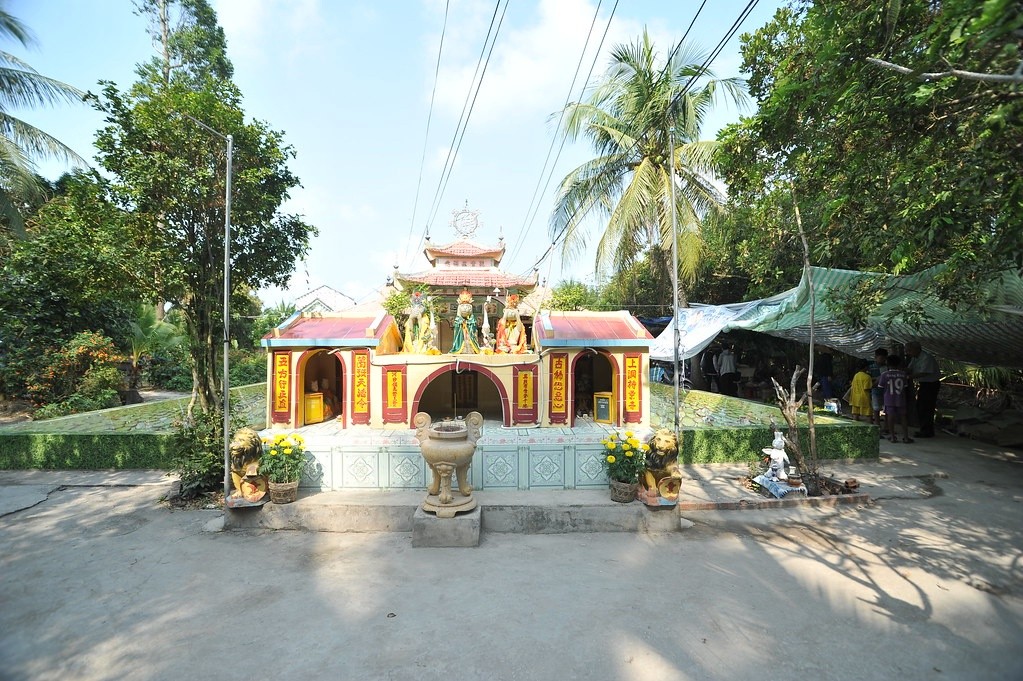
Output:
[648,264,1023,373]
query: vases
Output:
[608,477,641,503]
[266,481,299,504]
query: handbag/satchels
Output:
[735,368,741,380]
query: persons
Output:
[404,290,528,353]
[699,340,940,443]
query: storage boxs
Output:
[304,393,324,424]
[593,391,613,424]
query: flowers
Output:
[255,433,308,484]
[600,432,653,486]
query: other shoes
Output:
[913,431,934,437]
[903,438,914,443]
[879,436,884,439]
[892,439,896,442]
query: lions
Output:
[635,428,683,503]
[229,426,266,501]
